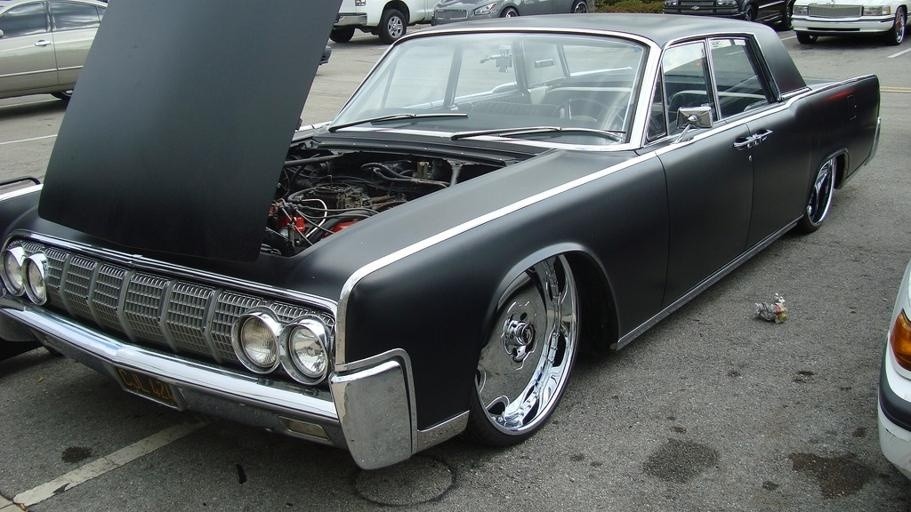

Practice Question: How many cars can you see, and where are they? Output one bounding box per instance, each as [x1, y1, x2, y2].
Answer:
[658, 0, 794, 29]
[428, 1, 597, 26]
[872, 252, 910, 487]
[329, 0, 436, 46]
[2, 1, 885, 476]
[0, 0, 109, 104]
[792, 1, 911, 48]
